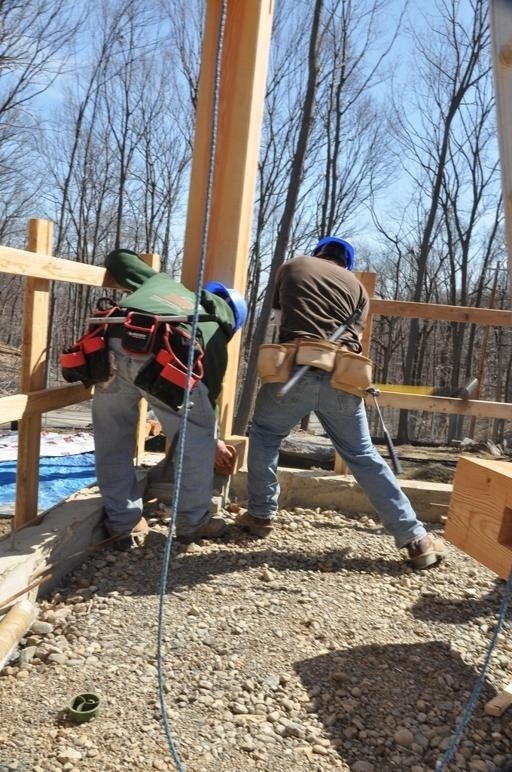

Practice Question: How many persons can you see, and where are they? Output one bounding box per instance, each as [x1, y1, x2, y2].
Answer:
[61, 249, 247, 549]
[232, 236, 441, 572]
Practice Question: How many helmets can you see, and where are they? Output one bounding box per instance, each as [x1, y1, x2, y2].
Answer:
[310, 235, 356, 270]
[206, 281, 249, 333]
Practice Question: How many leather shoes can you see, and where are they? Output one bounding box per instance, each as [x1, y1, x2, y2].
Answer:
[234, 512, 275, 537]
[114, 517, 149, 550]
[180, 518, 228, 544]
[408, 536, 446, 569]
